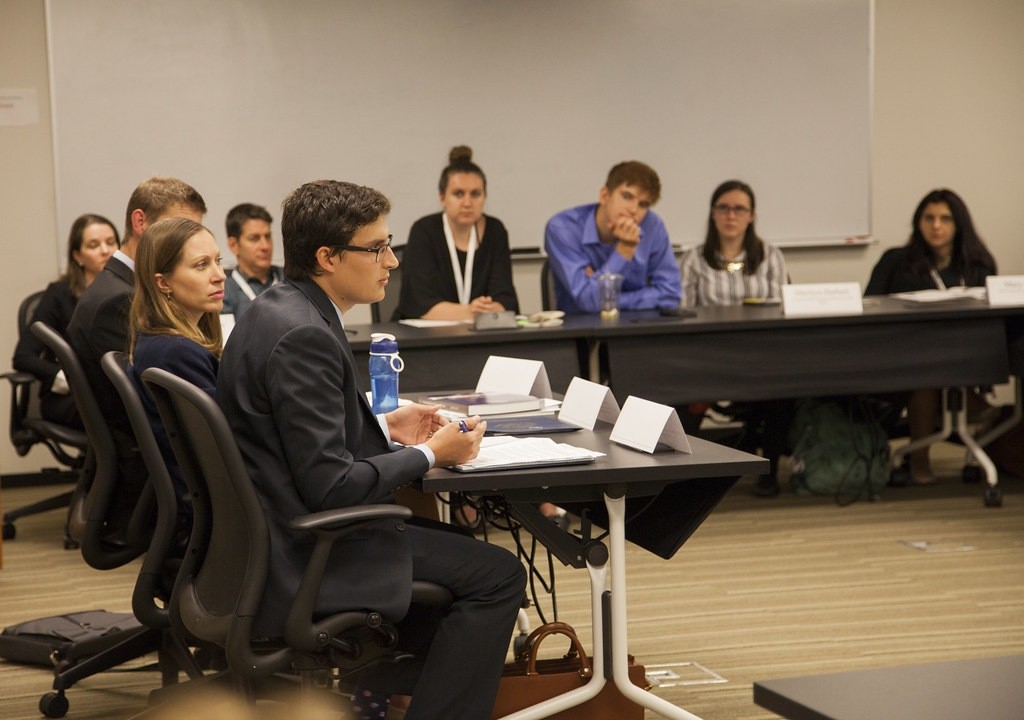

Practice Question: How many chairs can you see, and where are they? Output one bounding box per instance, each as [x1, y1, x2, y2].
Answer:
[1, 287, 452, 720]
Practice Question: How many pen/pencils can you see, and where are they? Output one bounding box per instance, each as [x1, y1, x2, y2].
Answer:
[459, 421, 468, 433]
[345, 329, 357, 335]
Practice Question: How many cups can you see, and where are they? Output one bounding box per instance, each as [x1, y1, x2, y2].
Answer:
[599, 274, 623, 321]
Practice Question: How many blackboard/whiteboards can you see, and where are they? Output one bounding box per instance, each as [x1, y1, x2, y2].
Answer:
[46, 0, 874, 277]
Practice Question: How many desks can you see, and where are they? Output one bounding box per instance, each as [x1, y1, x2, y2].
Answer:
[371, 387, 770, 720]
[339, 289, 1023, 506]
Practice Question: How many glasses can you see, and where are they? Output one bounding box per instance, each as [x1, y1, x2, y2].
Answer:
[326, 235, 393, 263]
[713, 203, 749, 217]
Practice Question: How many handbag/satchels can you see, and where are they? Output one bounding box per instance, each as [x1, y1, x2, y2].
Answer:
[1, 610, 145, 667]
[490, 622, 646, 720]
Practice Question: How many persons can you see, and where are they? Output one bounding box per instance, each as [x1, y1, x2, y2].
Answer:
[219, 205, 287, 326]
[861, 189, 1019, 486]
[217, 179, 527, 720]
[543, 161, 682, 313]
[679, 179, 792, 497]
[64, 177, 207, 519]
[12, 214, 121, 431]
[129, 216, 227, 403]
[393, 144, 519, 323]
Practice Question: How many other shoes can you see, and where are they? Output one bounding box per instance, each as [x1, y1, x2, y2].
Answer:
[755, 480, 779, 498]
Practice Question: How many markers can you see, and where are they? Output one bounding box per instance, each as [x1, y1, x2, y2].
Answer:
[846, 234, 870, 242]
[672, 244, 696, 249]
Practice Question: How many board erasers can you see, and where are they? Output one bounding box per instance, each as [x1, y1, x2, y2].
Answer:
[510, 248, 540, 255]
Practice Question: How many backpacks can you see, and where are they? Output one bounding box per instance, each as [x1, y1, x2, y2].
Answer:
[789, 400, 893, 504]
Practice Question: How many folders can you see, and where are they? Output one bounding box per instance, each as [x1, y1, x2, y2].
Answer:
[475, 415, 583, 437]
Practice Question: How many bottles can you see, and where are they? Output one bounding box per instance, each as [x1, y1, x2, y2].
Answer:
[368, 332, 405, 416]
